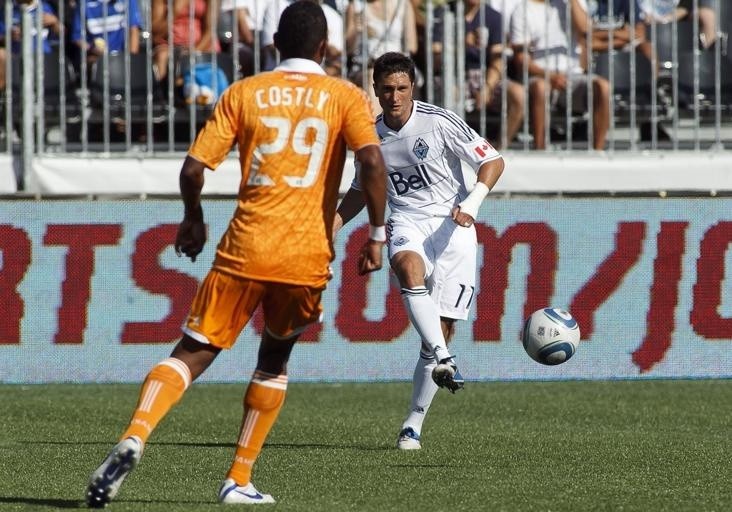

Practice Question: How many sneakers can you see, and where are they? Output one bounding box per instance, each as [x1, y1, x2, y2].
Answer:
[84, 435, 144, 508]
[219, 479, 275, 504]
[431, 356, 465, 393]
[396, 427, 421, 450]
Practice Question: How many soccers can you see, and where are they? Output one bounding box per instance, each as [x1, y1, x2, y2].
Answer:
[523, 308, 580, 366]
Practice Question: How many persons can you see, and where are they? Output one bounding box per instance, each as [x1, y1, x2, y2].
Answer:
[333, 50, 508, 450]
[572, 0, 732, 86]
[86, 2, 391, 509]
[1, 0, 611, 150]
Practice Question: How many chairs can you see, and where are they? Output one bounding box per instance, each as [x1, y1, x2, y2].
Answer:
[0, 22, 732, 147]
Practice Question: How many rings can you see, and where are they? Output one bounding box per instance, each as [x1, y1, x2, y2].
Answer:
[463, 220, 471, 228]
